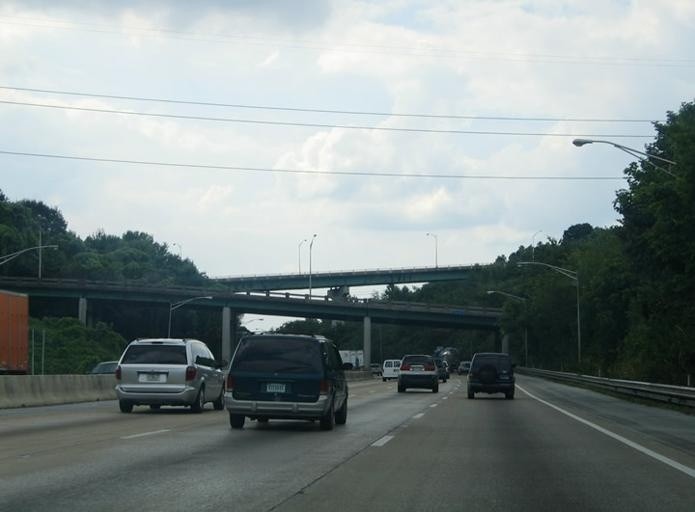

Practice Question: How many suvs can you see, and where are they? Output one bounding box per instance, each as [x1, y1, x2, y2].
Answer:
[371, 346, 519, 400]
[222, 328, 351, 429]
[112, 336, 226, 414]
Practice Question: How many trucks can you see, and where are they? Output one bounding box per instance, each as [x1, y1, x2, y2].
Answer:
[338, 347, 365, 371]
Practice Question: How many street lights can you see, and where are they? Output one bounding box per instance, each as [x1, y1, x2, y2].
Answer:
[1, 242, 62, 267]
[569, 137, 695, 206]
[167, 295, 213, 337]
[244, 318, 264, 325]
[517, 259, 586, 375]
[308, 232, 318, 300]
[173, 242, 183, 259]
[424, 231, 438, 268]
[297, 238, 307, 275]
[485, 289, 530, 368]
[532, 228, 543, 262]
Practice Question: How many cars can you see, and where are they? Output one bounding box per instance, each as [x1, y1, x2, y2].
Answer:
[88, 360, 121, 374]
